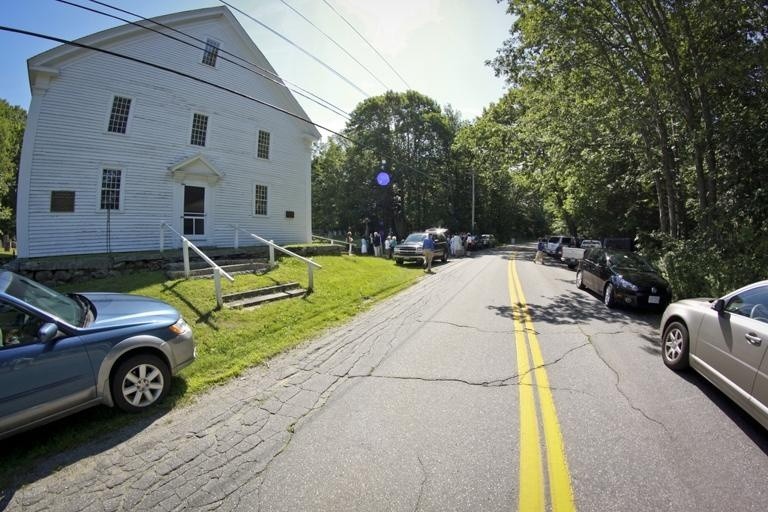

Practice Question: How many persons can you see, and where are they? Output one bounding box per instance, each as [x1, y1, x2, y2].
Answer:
[361, 232, 397, 258]
[447, 231, 475, 258]
[345, 231, 353, 254]
[533, 238, 543, 266]
[422, 233, 434, 271]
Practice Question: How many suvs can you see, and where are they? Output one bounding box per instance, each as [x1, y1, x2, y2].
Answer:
[393, 232, 450, 266]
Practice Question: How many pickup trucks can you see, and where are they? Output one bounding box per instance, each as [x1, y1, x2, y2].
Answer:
[561, 239, 603, 269]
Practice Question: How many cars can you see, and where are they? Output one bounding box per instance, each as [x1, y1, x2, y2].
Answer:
[603, 237, 634, 250]
[481, 234, 497, 248]
[659, 280, 768, 430]
[575, 247, 672, 310]
[538, 235, 574, 257]
[0, 268, 196, 440]
